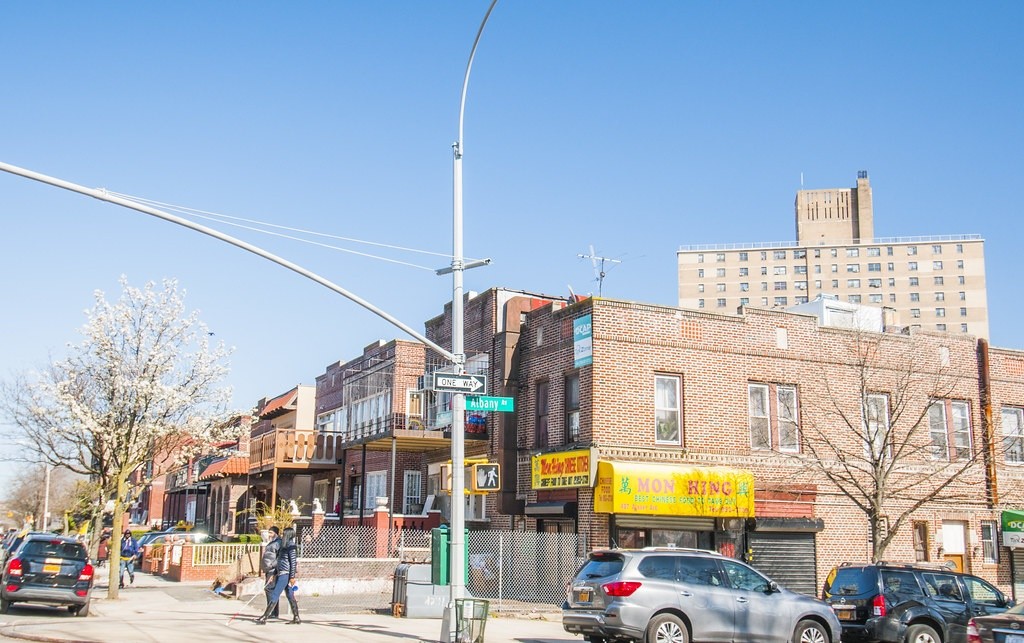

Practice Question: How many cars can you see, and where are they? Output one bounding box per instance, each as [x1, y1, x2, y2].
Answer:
[133, 520, 225, 568]
[967, 602, 1024, 643]
[0, 528, 54, 571]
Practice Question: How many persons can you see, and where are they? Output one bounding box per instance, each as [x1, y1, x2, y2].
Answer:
[259, 526, 282, 618]
[252, 528, 301, 625]
[940, 584, 961, 599]
[97, 536, 108, 566]
[118, 530, 139, 588]
[334, 496, 341, 516]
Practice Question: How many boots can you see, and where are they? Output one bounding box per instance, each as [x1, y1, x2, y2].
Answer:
[285, 599, 301, 624]
[252, 601, 277, 625]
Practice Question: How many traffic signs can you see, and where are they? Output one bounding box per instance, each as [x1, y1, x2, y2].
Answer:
[433, 371, 488, 395]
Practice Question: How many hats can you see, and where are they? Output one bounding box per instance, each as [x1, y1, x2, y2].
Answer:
[125, 530, 131, 534]
[269, 526, 279, 536]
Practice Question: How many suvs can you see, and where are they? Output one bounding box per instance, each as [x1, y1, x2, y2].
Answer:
[0, 534, 95, 618]
[821, 562, 1020, 643]
[561, 545, 845, 643]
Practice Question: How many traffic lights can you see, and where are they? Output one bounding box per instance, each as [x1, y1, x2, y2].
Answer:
[472, 464, 503, 494]
[441, 464, 452, 494]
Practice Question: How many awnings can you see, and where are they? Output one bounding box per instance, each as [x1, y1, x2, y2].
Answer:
[1001, 510, 1024, 547]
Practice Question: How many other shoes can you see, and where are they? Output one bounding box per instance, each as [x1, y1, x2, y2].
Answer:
[269, 613, 279, 619]
[130, 574, 134, 583]
[119, 583, 124, 589]
